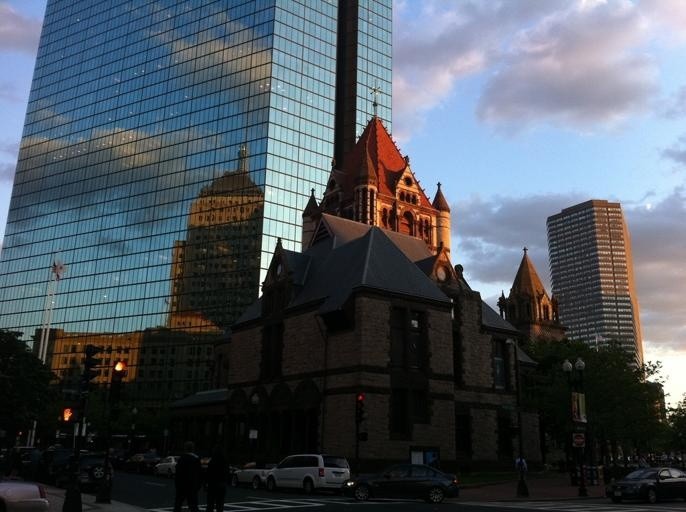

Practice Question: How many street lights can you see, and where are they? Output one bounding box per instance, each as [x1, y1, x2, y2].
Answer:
[561, 356, 588, 496]
[504, 336, 530, 498]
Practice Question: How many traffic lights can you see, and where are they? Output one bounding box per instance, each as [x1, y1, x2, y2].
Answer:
[62, 407, 80, 424]
[355, 393, 365, 424]
[85, 343, 101, 380]
[109, 359, 128, 404]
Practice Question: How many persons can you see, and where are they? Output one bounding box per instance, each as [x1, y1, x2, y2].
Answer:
[169, 438, 204, 510]
[199, 439, 228, 511]
[514, 456, 527, 480]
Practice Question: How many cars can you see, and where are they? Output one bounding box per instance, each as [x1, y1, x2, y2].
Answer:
[0, 480, 51, 512]
[231, 454, 460, 504]
[13, 446, 106, 487]
[607, 467, 686, 504]
[125, 452, 184, 479]
